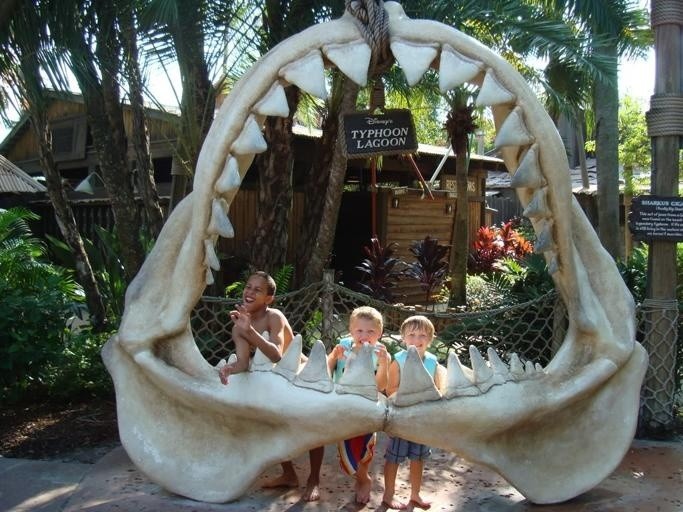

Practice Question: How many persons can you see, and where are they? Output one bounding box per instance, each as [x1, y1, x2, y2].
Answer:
[386, 316, 447, 509]
[219, 269, 324, 501]
[328, 306, 391, 504]
